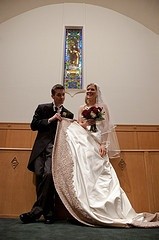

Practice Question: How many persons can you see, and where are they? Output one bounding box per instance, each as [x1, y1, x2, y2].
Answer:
[78, 82, 109, 226]
[20, 84, 74, 224]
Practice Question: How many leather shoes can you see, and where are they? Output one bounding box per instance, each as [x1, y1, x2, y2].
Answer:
[44, 217, 56, 224]
[19, 212, 39, 223]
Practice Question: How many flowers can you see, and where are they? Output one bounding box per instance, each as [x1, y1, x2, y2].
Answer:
[81, 106, 105, 132]
